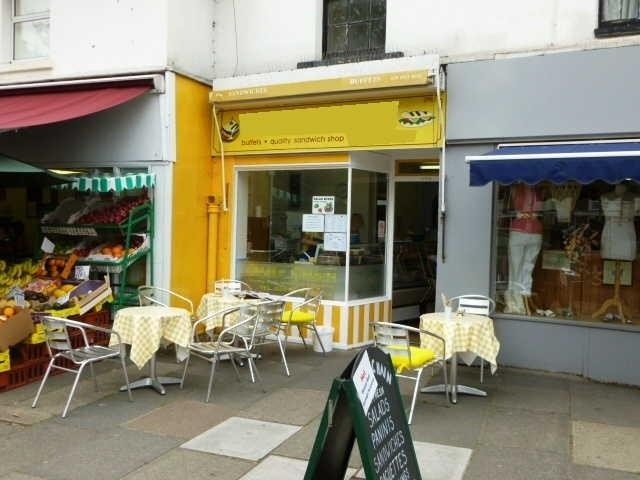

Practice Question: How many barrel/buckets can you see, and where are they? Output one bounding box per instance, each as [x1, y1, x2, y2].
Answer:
[313, 327, 333, 353]
[350, 248, 369, 265]
[316, 255, 340, 265]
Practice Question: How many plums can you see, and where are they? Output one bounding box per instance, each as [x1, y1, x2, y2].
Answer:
[114, 235, 144, 249]
[74, 240, 104, 257]
[8, 290, 47, 302]
[75, 195, 143, 224]
[30, 312, 53, 325]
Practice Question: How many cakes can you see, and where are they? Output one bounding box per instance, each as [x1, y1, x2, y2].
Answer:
[221, 115, 240, 142]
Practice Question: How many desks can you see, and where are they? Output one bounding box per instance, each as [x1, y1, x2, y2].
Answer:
[413, 312, 503, 405]
[193, 293, 270, 368]
[105, 306, 195, 398]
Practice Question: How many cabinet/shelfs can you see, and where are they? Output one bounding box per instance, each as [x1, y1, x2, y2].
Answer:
[348, 240, 432, 324]
[38, 191, 157, 324]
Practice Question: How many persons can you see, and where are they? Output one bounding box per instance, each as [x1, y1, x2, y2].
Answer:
[505, 184, 546, 295]
[427, 255, 437, 263]
[601, 183, 640, 261]
[302, 233, 323, 261]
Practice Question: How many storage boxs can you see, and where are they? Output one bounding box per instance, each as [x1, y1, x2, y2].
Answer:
[0, 277, 112, 354]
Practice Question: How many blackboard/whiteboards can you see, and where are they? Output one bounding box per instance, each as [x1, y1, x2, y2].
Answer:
[342, 348, 423, 480]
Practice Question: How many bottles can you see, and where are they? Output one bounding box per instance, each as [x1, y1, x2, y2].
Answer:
[255, 204, 263, 217]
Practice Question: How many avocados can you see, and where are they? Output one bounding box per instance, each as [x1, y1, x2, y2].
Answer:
[34, 303, 62, 311]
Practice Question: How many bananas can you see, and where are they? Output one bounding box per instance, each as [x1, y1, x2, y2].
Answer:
[0, 257, 40, 298]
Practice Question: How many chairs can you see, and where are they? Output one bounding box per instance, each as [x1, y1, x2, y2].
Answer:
[178, 303, 264, 404]
[137, 284, 198, 318]
[223, 299, 291, 381]
[272, 285, 327, 377]
[212, 278, 266, 355]
[368, 318, 452, 428]
[440, 293, 497, 385]
[30, 316, 135, 420]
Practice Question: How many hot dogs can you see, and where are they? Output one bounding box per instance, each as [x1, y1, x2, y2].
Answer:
[399, 111, 434, 127]
